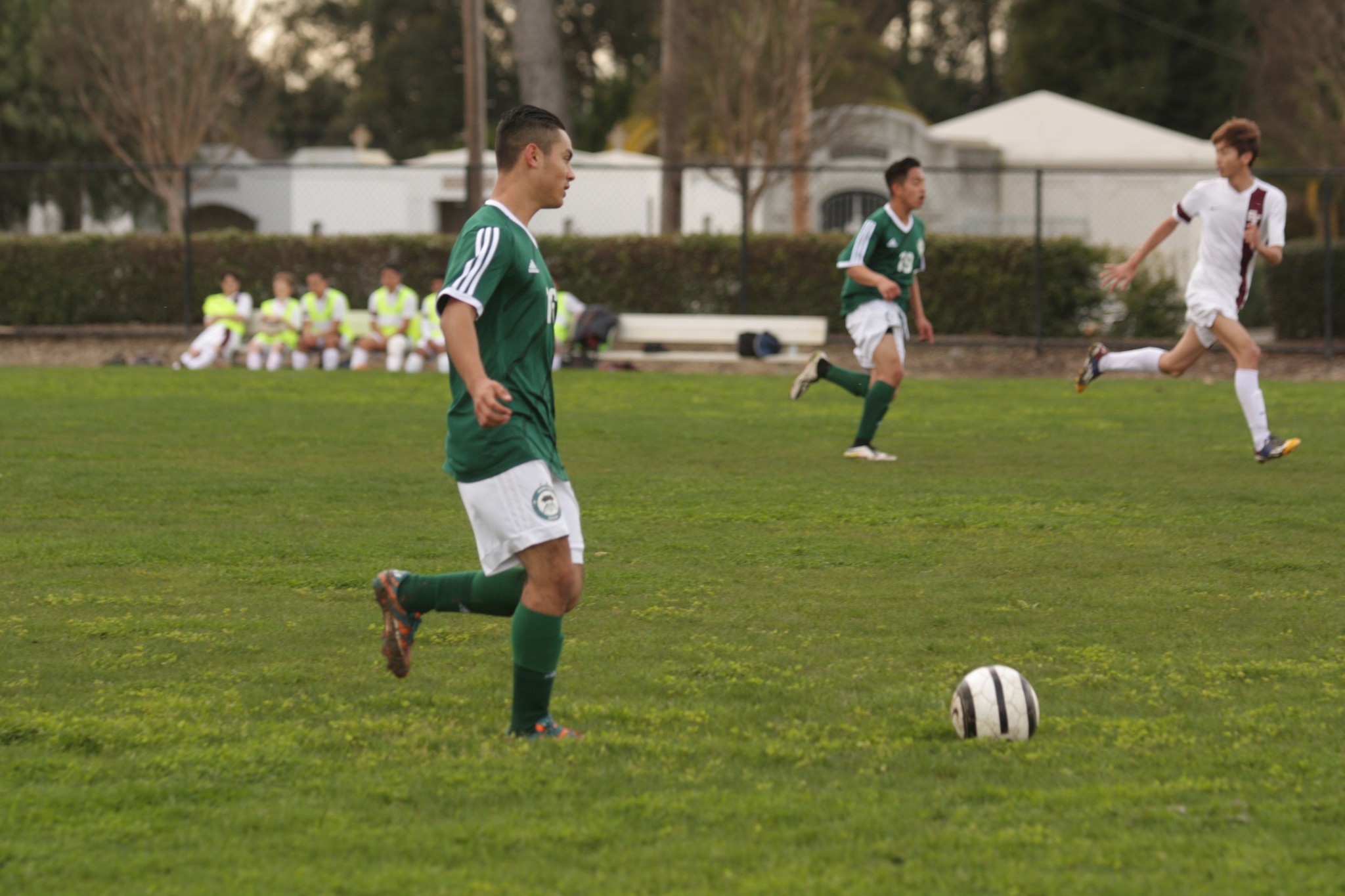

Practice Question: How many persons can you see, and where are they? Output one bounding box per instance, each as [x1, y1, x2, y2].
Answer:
[788, 156, 934, 456]
[372, 103, 586, 740]
[171, 263, 450, 373]
[551, 275, 585, 371]
[1075, 118, 1302, 464]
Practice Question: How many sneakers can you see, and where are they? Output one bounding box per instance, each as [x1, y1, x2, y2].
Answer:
[1076, 342, 1110, 391]
[1255, 435, 1302, 463]
[373, 568, 421, 677]
[791, 351, 830, 400]
[534, 715, 583, 739]
[843, 445, 898, 462]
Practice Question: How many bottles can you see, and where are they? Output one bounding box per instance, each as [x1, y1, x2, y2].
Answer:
[171, 361, 182, 370]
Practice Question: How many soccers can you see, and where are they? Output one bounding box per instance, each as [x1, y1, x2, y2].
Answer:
[950, 664, 1040, 743]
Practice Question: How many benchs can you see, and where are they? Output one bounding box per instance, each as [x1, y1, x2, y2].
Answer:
[220, 306, 829, 377]
